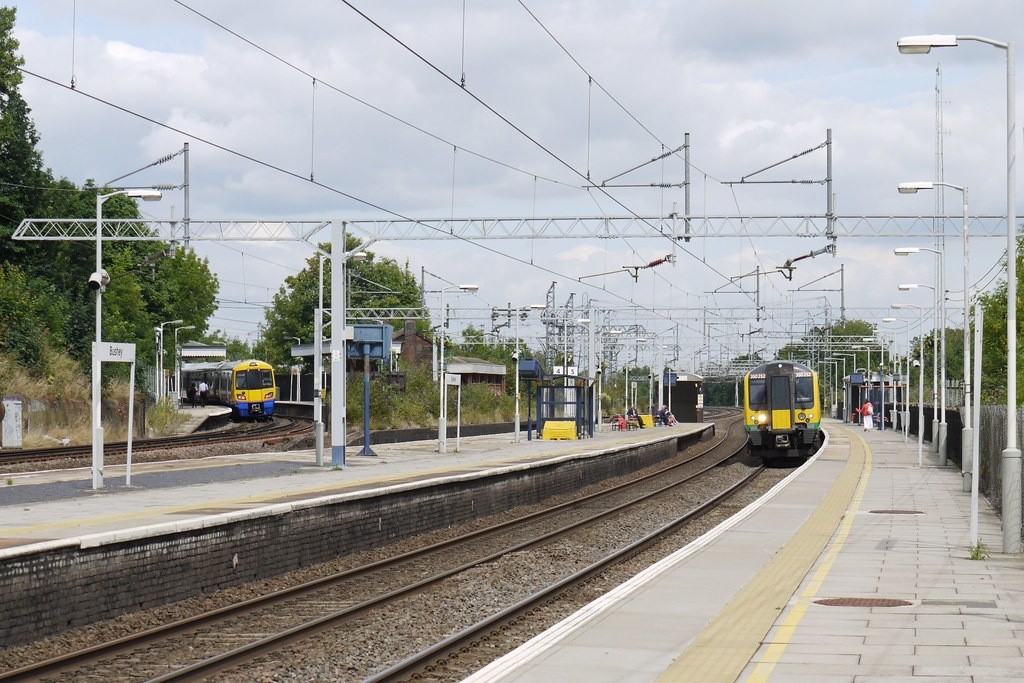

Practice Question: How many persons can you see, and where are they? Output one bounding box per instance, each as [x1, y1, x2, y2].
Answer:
[857, 399, 874, 432]
[188, 383, 198, 408]
[664, 407, 679, 425]
[627, 404, 646, 429]
[198, 380, 208, 407]
[658, 405, 673, 426]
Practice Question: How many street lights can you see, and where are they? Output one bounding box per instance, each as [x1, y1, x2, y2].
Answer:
[896, 283, 939, 455]
[564, 318, 590, 416]
[899, 178, 973, 496]
[818, 335, 897, 432]
[599, 330, 621, 431]
[92, 183, 164, 489]
[513, 303, 546, 442]
[624, 338, 646, 421]
[315, 251, 368, 465]
[437, 282, 479, 451]
[891, 302, 926, 447]
[873, 328, 906, 436]
[895, 244, 949, 468]
[898, 29, 1023, 552]
[883, 317, 911, 439]
[648, 344, 669, 415]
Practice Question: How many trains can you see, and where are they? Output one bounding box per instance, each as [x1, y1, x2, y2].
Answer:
[181, 359, 277, 420]
[743, 359, 822, 468]
